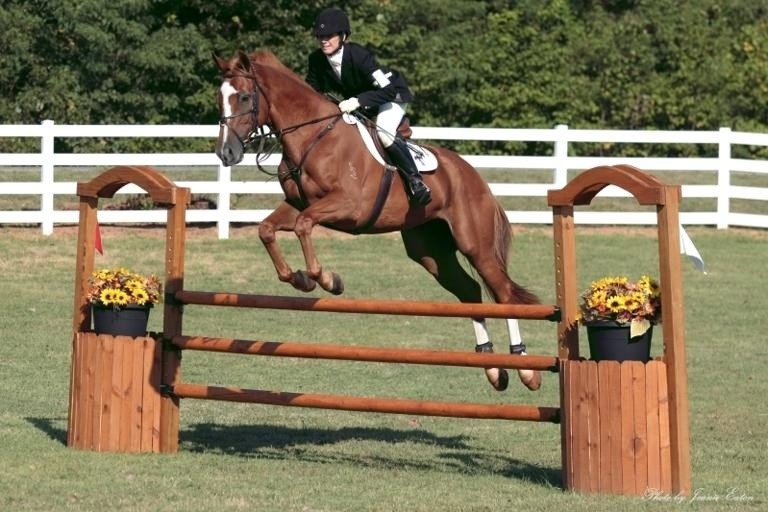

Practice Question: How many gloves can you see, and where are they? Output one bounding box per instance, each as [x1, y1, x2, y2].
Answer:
[339, 97, 360, 114]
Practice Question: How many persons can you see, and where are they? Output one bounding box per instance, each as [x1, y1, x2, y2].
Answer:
[303, 8, 433, 211]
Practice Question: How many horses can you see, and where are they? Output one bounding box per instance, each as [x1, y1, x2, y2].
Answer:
[211, 47, 543, 391]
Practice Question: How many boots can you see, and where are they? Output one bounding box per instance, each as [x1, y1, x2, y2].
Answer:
[386, 135, 432, 208]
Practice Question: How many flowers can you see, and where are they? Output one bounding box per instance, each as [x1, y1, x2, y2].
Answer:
[575, 274, 663, 329]
[84, 264, 162, 310]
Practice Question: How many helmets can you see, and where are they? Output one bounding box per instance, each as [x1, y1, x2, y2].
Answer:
[311, 8, 350, 36]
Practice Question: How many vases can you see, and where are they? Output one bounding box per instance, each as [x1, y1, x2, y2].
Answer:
[584, 319, 654, 366]
[91, 301, 151, 341]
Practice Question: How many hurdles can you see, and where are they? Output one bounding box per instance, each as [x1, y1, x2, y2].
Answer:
[66, 165, 692, 498]
